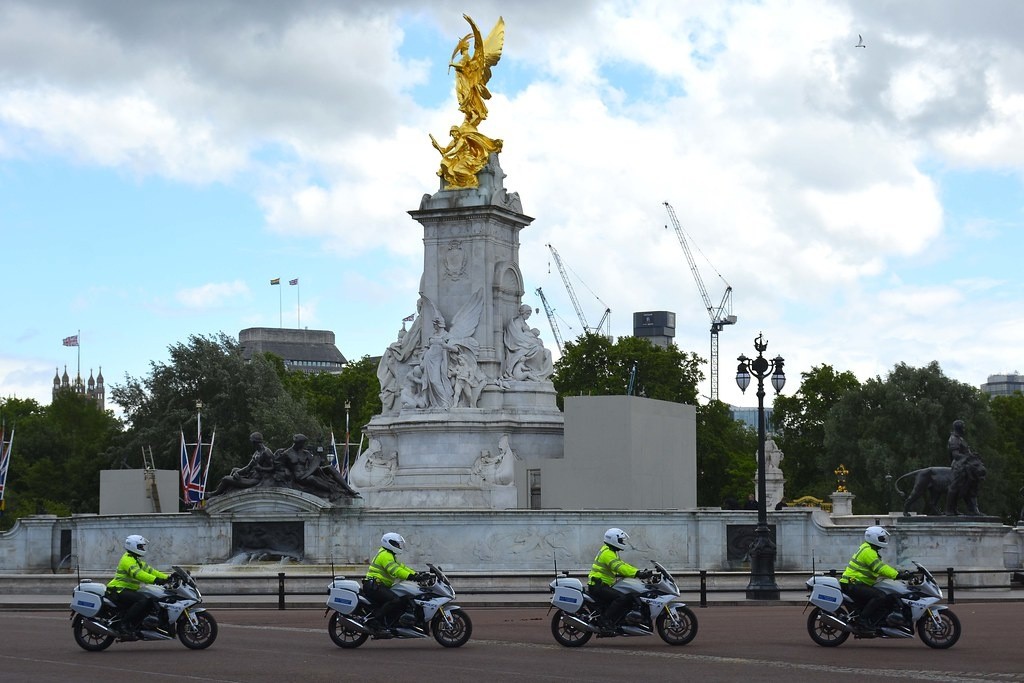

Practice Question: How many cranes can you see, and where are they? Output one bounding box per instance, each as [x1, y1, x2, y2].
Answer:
[545, 242, 610, 342]
[535, 287, 579, 358]
[664, 201, 737, 401]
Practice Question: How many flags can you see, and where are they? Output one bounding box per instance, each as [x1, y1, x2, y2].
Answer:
[63, 335, 79, 346]
[270, 278, 280, 285]
[0, 430, 12, 500]
[329, 415, 364, 486]
[289, 279, 299, 285]
[181, 430, 215, 506]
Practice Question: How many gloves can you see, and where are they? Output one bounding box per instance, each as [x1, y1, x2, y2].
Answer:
[408, 573, 423, 581]
[154, 577, 170, 585]
[896, 572, 914, 580]
[638, 568, 653, 578]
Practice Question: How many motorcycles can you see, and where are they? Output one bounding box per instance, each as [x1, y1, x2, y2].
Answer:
[803, 549, 961, 649]
[69, 555, 218, 653]
[546, 551, 699, 648]
[324, 554, 473, 649]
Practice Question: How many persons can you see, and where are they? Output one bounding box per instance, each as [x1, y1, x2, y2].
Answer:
[432, 126, 502, 190]
[449, 14, 505, 129]
[765, 432, 778, 468]
[378, 299, 474, 408]
[588, 527, 646, 630]
[949, 419, 979, 491]
[106, 535, 171, 635]
[272, 434, 362, 498]
[363, 533, 424, 629]
[349, 440, 398, 488]
[208, 432, 274, 498]
[471, 435, 514, 486]
[504, 305, 553, 381]
[839, 527, 909, 632]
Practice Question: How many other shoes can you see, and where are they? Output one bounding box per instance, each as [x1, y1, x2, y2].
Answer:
[615, 625, 630, 634]
[116, 623, 133, 635]
[370, 622, 387, 630]
[854, 623, 875, 631]
[599, 621, 618, 630]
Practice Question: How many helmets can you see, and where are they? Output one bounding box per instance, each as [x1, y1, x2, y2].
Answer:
[604, 527, 630, 551]
[865, 526, 891, 548]
[123, 534, 149, 557]
[380, 532, 404, 554]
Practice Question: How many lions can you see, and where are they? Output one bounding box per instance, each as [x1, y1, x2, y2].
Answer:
[893, 452, 989, 517]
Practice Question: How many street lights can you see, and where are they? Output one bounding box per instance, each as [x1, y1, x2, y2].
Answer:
[735, 330, 787, 600]
[886, 472, 893, 512]
